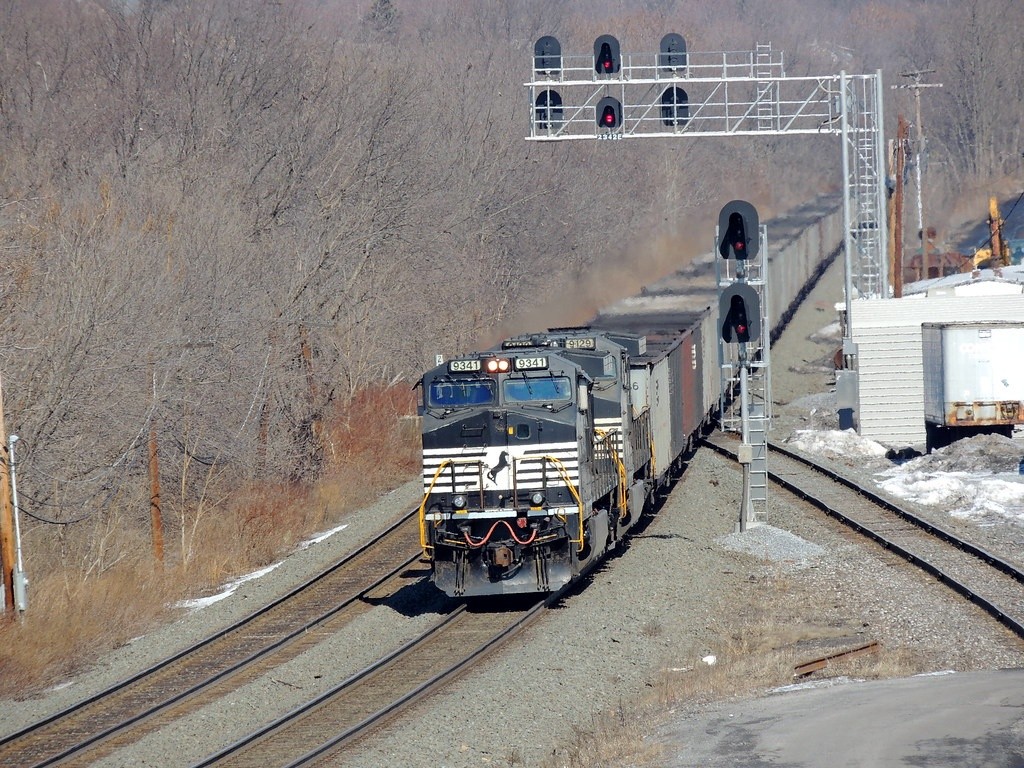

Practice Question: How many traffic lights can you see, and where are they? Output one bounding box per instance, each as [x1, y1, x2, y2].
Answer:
[716, 199, 759, 260]
[718, 283, 761, 343]
[597, 97, 622, 128]
[593, 34, 620, 74]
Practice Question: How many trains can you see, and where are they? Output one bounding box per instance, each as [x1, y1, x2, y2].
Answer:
[412, 171, 888, 602]
